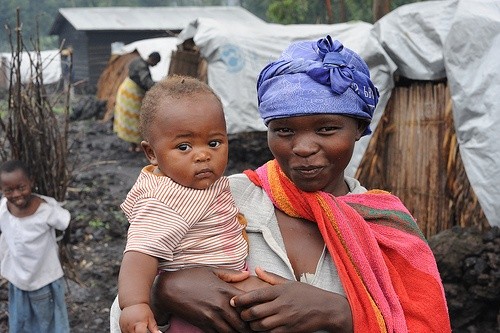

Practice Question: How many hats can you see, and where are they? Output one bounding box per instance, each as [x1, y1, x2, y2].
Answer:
[256, 35, 379, 136]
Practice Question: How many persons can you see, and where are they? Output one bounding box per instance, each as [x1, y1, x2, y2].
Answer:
[115, 74, 287, 333]
[113, 52, 162, 152]
[0, 159, 72, 333]
[116, 37, 452, 333]
